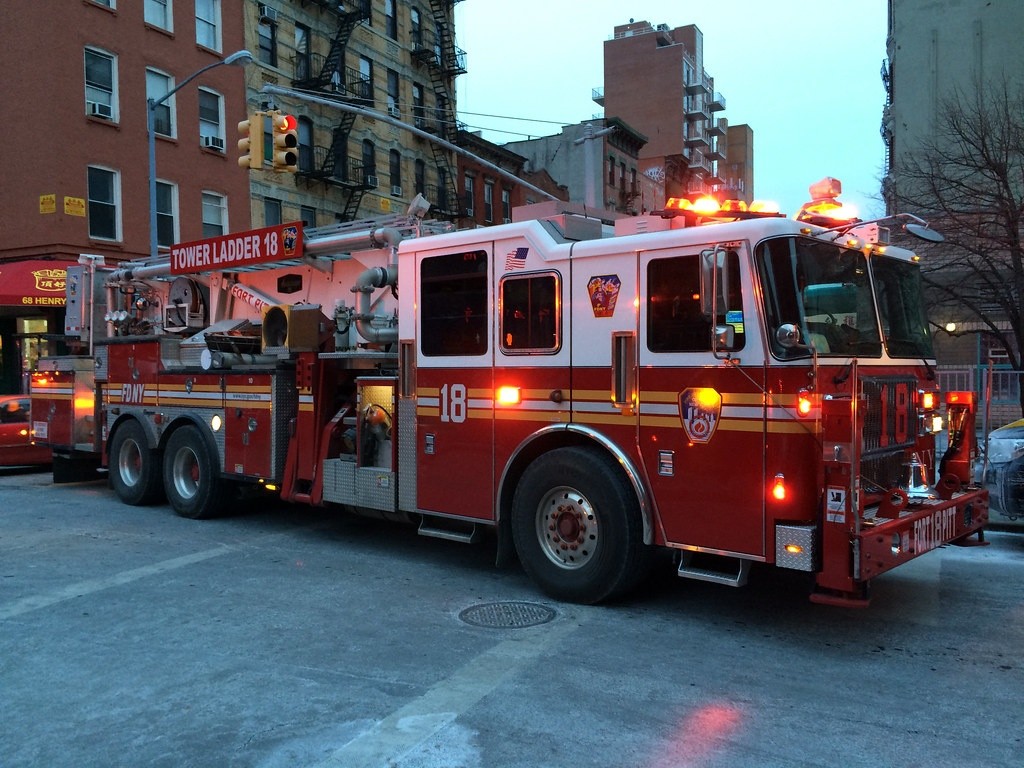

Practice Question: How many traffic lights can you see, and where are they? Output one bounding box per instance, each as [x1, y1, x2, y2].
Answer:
[237, 113, 262, 171]
[272, 112, 300, 173]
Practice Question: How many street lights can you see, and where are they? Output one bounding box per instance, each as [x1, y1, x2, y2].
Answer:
[146, 49, 254, 256]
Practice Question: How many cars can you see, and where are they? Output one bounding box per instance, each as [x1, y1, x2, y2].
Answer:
[978, 426, 1024, 520]
[0, 396, 58, 465]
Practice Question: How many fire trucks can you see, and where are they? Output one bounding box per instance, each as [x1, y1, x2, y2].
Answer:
[30, 184, 991, 617]
